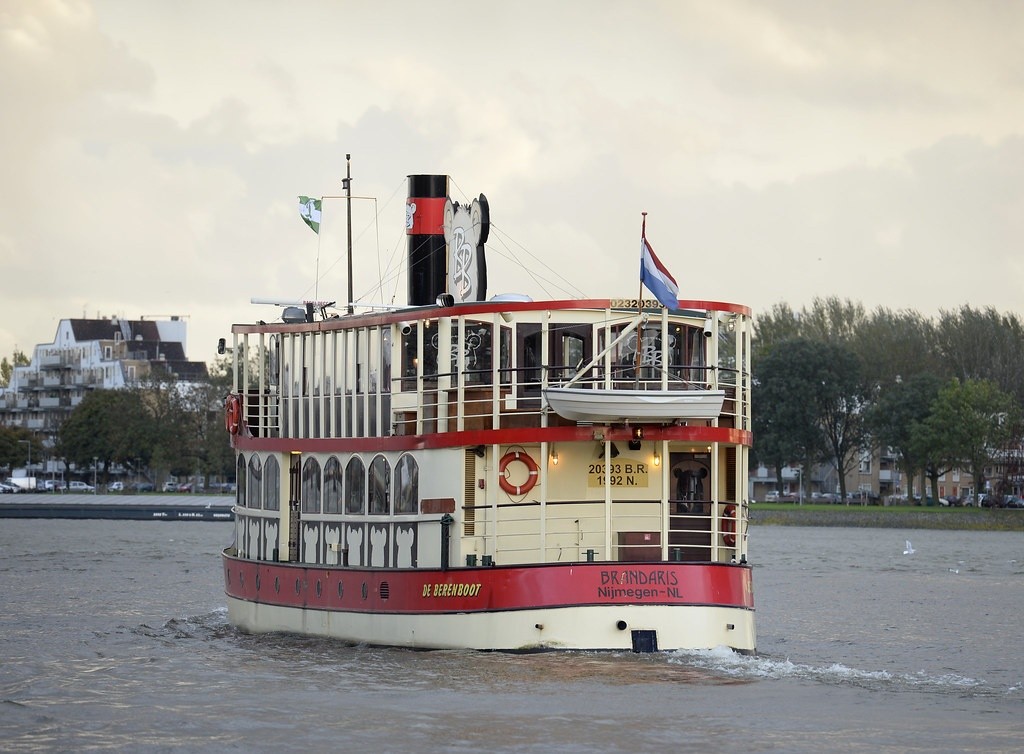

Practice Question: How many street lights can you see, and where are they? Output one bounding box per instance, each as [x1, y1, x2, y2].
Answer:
[93, 457, 99, 495]
[134, 458, 142, 494]
[18, 439, 31, 492]
[61, 456, 65, 494]
[52, 456, 57, 493]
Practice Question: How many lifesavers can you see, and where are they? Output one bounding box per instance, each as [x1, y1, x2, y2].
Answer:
[223, 394, 240, 436]
[498, 452, 539, 496]
[721, 504, 736, 547]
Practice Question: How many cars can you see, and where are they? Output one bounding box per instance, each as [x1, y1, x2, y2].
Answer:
[0, 476, 96, 495]
[137, 482, 156, 492]
[107, 481, 124, 492]
[162, 476, 235, 493]
[765, 487, 1024, 508]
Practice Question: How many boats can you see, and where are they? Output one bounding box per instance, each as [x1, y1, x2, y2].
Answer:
[541, 383, 725, 425]
[221, 154, 760, 659]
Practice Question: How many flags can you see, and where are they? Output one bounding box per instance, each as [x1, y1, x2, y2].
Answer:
[640, 220, 680, 311]
[298, 195, 322, 234]
